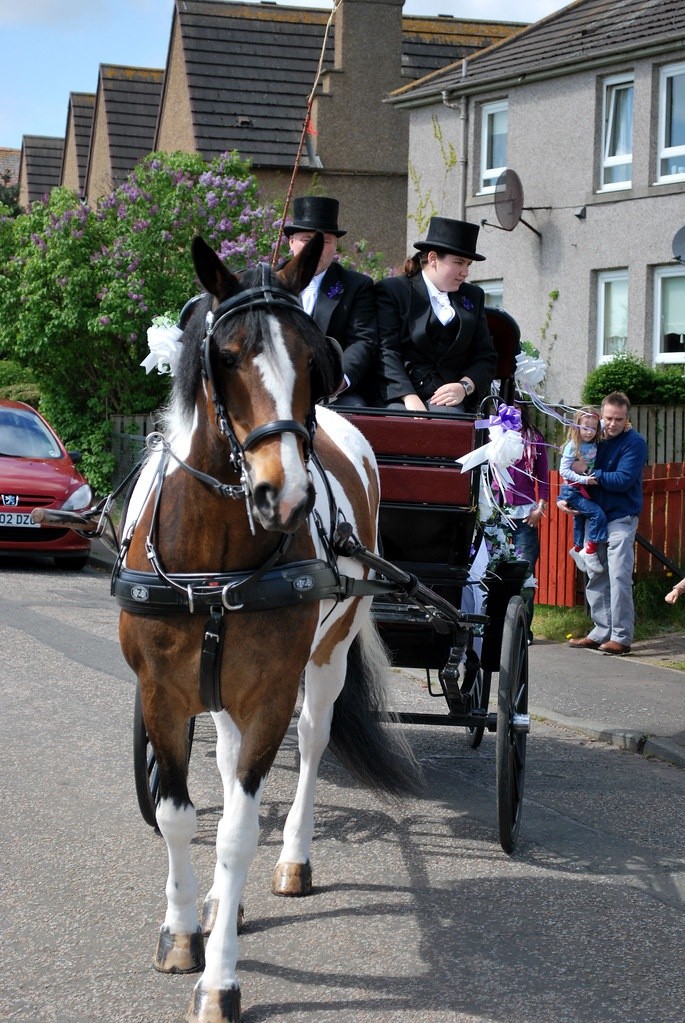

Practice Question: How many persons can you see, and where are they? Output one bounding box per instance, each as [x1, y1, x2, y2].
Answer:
[557, 391, 646, 654]
[255, 194, 378, 411]
[664, 578, 684, 605]
[371, 216, 494, 421]
[560, 407, 632, 571]
[484, 393, 548, 645]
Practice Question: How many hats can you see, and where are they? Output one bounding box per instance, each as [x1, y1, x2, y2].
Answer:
[413, 217, 487, 261]
[283, 196, 347, 239]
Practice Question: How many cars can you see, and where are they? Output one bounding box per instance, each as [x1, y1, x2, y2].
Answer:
[0, 397, 98, 572]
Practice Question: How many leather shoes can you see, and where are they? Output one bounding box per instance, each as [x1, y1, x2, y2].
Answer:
[569, 636, 601, 648]
[598, 641, 630, 654]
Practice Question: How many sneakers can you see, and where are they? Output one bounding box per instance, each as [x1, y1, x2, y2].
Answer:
[569, 546, 587, 572]
[579, 547, 603, 573]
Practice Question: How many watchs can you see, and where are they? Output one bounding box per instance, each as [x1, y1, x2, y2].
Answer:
[458, 380, 472, 395]
[538, 502, 547, 510]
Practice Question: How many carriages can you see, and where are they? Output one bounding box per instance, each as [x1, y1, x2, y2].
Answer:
[28, 229, 542, 1023]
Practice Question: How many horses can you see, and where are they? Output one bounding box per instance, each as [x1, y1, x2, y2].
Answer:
[118, 234, 380, 1023]
